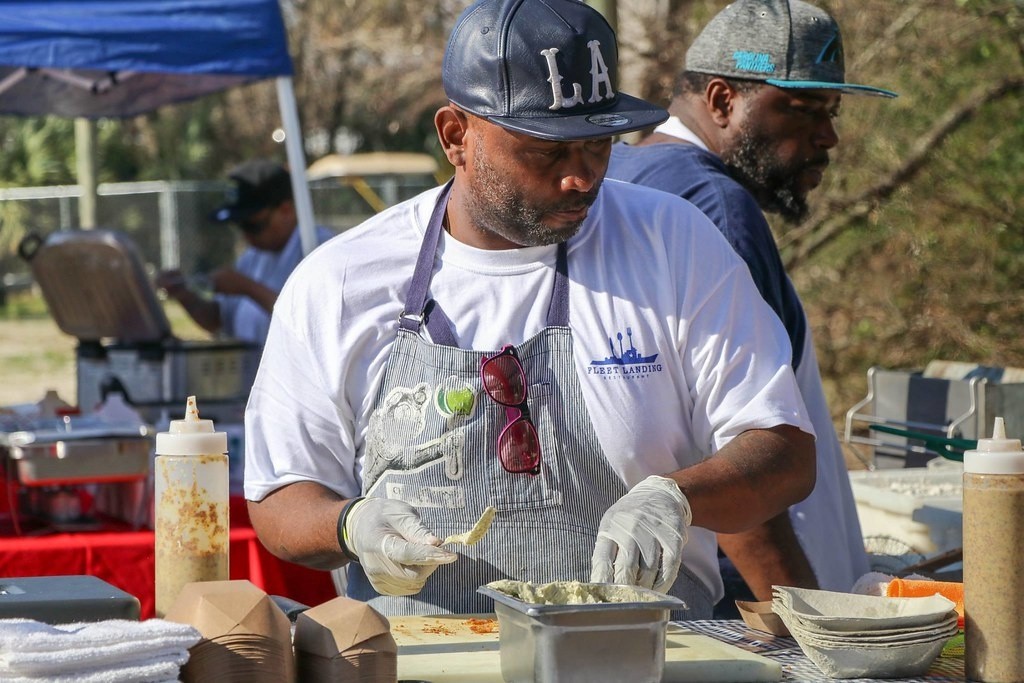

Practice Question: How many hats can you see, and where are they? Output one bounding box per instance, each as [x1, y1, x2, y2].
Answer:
[440, 0, 672, 142]
[683, 1, 898, 99]
[230, 161, 293, 213]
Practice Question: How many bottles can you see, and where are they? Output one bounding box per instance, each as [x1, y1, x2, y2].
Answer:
[962, 414, 1024, 683]
[154, 396, 228, 619]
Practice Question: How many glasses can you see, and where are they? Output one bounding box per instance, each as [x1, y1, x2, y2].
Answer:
[229, 210, 274, 233]
[481, 344, 542, 478]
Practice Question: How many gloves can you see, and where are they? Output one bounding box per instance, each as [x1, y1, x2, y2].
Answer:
[590, 474, 694, 595]
[347, 496, 459, 598]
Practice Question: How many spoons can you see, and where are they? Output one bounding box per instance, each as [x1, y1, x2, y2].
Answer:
[437, 507, 495, 547]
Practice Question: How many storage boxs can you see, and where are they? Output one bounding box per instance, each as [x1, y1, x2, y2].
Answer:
[18, 228, 261, 429]
[847, 466, 963, 554]
[0, 430, 152, 538]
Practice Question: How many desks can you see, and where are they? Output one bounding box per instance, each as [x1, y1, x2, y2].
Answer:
[0, 492, 337, 608]
[150, 614, 965, 683]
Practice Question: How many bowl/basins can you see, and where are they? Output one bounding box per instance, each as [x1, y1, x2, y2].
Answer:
[770, 584, 960, 680]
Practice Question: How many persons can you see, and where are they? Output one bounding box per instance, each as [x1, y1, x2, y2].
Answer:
[240, 0, 821, 624]
[601, 2, 877, 625]
[153, 160, 336, 347]
